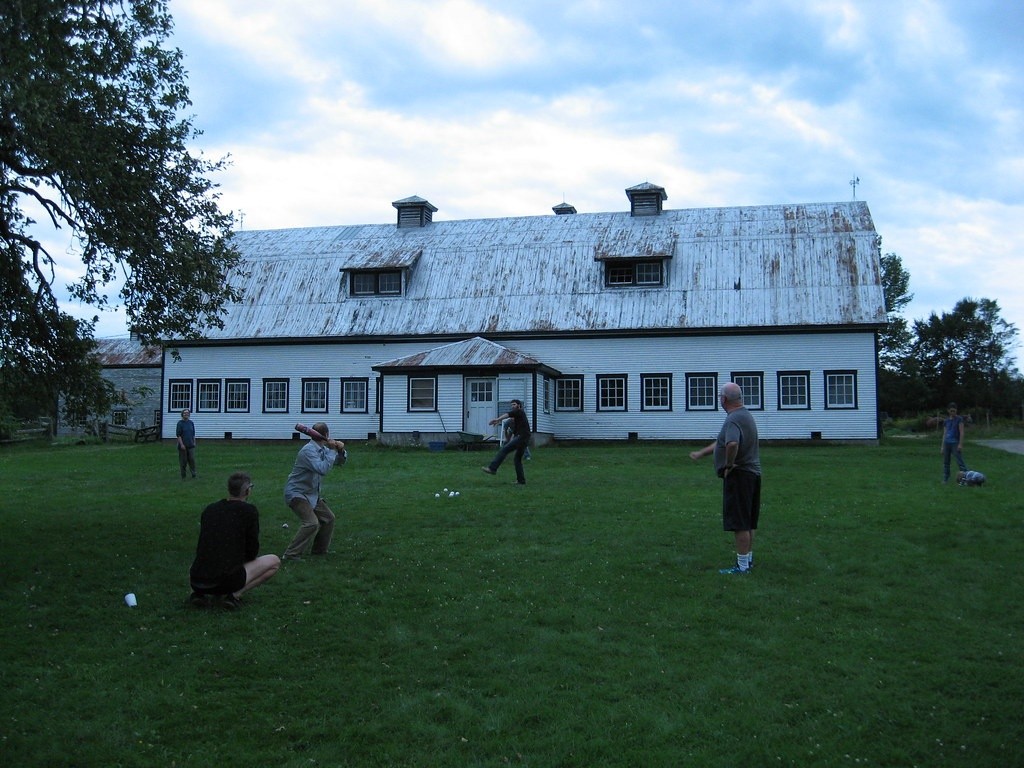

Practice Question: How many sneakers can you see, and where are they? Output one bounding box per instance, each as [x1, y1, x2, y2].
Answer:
[190, 591, 208, 605]
[219, 593, 238, 606]
[720, 565, 751, 575]
[481, 467, 496, 475]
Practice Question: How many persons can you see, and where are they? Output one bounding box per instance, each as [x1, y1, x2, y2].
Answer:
[481, 399, 532, 485]
[503, 412, 532, 461]
[689, 381, 763, 576]
[955, 471, 986, 488]
[189, 473, 283, 612]
[175, 408, 197, 483]
[281, 421, 348, 564]
[938, 402, 968, 487]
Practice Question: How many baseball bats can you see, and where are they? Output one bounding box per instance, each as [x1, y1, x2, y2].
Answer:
[294, 423, 341, 447]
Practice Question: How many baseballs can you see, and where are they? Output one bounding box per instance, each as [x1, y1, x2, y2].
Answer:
[282, 523, 288, 529]
[450, 491, 454, 496]
[448, 494, 453, 499]
[443, 488, 448, 492]
[455, 491, 461, 496]
[434, 493, 440, 498]
